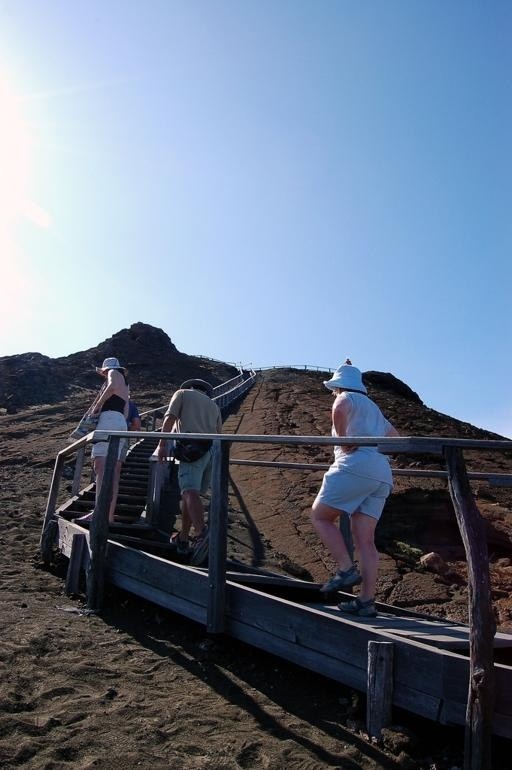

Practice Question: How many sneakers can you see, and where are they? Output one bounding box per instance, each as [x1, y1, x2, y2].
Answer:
[338, 596, 376, 616]
[319, 564, 363, 593]
[76, 510, 94, 521]
[170, 532, 189, 554]
[190, 527, 209, 566]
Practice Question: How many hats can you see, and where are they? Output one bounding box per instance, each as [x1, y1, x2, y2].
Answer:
[96, 357, 129, 376]
[323, 364, 368, 394]
[179, 378, 213, 395]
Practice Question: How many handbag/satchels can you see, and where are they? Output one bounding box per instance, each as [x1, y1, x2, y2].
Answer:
[174, 439, 213, 463]
[69, 410, 99, 439]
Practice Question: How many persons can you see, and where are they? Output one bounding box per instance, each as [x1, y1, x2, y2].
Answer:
[70, 357, 130, 524]
[156, 377, 223, 566]
[113, 398, 141, 514]
[309, 363, 403, 618]
